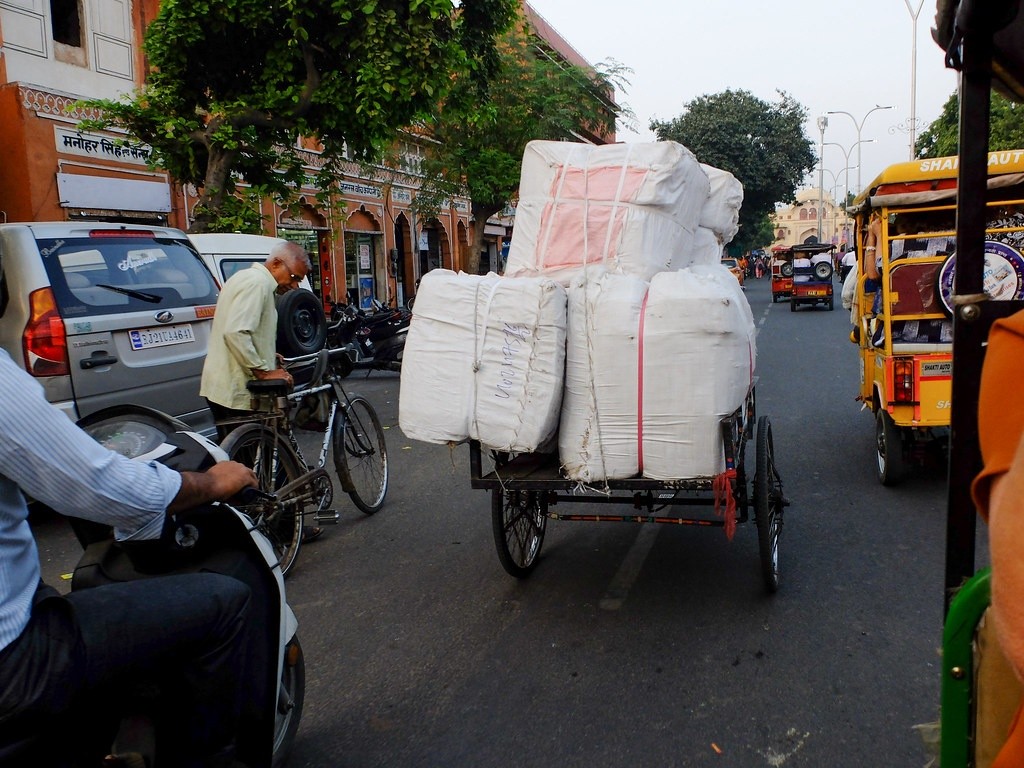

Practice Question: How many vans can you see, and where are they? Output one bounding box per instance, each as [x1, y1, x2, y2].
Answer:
[59, 231, 327, 385]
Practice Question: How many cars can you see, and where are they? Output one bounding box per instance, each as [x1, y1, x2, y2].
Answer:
[1, 220, 226, 451]
[721, 257, 744, 286]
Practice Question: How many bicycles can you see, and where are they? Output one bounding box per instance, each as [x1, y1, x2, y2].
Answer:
[209, 344, 388, 585]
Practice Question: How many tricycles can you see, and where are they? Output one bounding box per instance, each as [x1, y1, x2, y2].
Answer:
[469, 383, 785, 595]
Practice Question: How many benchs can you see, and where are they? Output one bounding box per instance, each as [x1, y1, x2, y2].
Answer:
[63, 268, 197, 306]
[791, 267, 832, 285]
[858, 272, 877, 351]
[772, 265, 792, 279]
[939, 566, 1024, 768]
[873, 253, 954, 356]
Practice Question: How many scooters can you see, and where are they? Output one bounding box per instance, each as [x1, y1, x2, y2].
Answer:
[325, 280, 423, 380]
[790, 242, 837, 311]
[16, 404, 306, 768]
[847, 149, 1024, 487]
[770, 245, 794, 303]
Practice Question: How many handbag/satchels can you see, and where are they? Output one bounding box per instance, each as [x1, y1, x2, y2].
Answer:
[758, 264, 763, 270]
[296, 349, 332, 431]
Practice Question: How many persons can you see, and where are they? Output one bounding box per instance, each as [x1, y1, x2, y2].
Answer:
[738, 251, 835, 283]
[835, 206, 1024, 349]
[0, 347, 276, 768]
[199, 240, 324, 549]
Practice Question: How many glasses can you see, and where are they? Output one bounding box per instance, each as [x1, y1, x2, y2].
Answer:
[278, 258, 302, 283]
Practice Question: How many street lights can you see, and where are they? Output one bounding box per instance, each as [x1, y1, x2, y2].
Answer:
[814, 106, 895, 242]
[817, 116, 828, 241]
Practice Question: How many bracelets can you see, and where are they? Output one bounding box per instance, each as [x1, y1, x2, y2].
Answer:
[866, 246, 876, 251]
